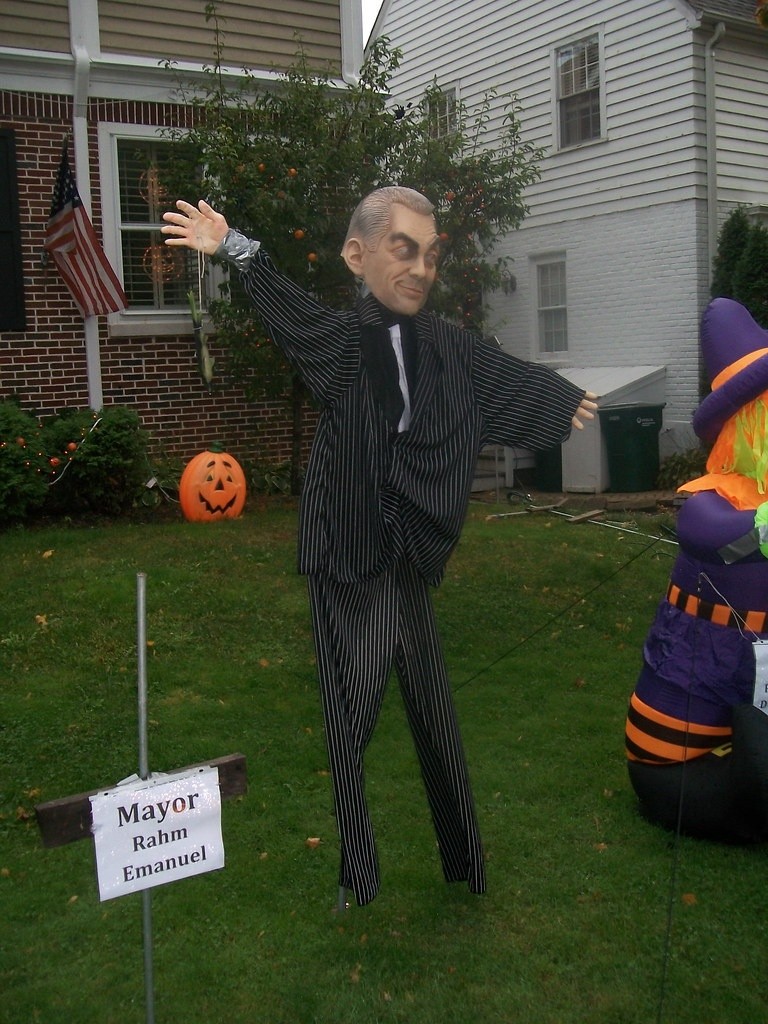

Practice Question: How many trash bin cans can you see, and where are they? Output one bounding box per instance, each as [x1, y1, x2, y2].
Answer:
[596, 401, 666, 494]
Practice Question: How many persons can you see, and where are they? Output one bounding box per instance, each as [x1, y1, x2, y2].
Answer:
[156, 185, 597, 915]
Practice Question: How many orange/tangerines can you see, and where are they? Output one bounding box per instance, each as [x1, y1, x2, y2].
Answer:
[236, 157, 317, 262]
[419, 172, 485, 242]
[16, 436, 79, 466]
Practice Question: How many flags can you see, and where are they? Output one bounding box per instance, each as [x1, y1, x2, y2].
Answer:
[45, 143, 128, 323]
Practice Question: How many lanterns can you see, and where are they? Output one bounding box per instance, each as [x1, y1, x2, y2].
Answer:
[177, 435, 249, 523]
